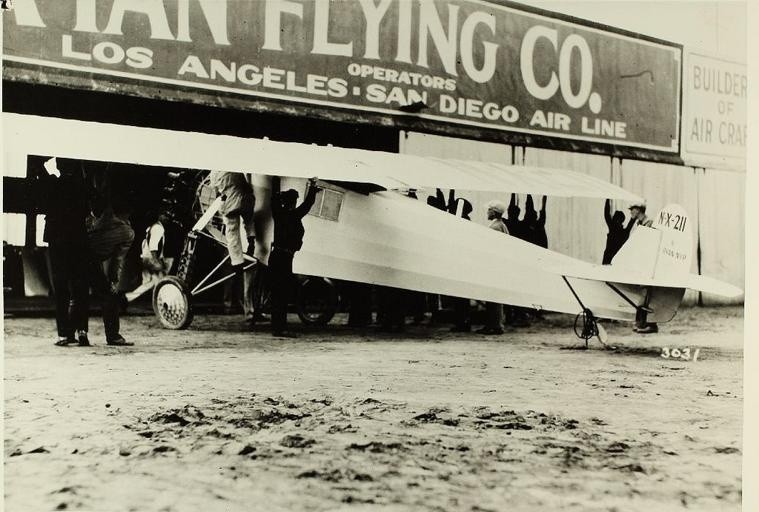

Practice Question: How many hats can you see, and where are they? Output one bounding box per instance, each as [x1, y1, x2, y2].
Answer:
[628, 201, 647, 209]
[486, 200, 505, 215]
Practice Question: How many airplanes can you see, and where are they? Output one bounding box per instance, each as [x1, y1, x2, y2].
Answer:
[2, 112, 743, 334]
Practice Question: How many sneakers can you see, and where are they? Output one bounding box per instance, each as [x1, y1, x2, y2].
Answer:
[632, 322, 659, 334]
[245, 245, 255, 256]
[234, 267, 244, 278]
[107, 336, 135, 346]
[350, 314, 547, 335]
[53, 330, 90, 346]
[272, 328, 297, 338]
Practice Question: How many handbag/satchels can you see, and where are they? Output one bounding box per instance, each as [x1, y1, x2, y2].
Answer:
[142, 255, 167, 273]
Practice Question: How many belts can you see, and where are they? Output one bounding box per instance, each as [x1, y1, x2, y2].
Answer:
[273, 246, 295, 256]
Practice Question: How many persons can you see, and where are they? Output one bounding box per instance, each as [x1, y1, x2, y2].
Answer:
[32, 155, 92, 346]
[267, 174, 323, 334]
[210, 170, 256, 281]
[68, 163, 141, 346]
[347, 185, 659, 333]
